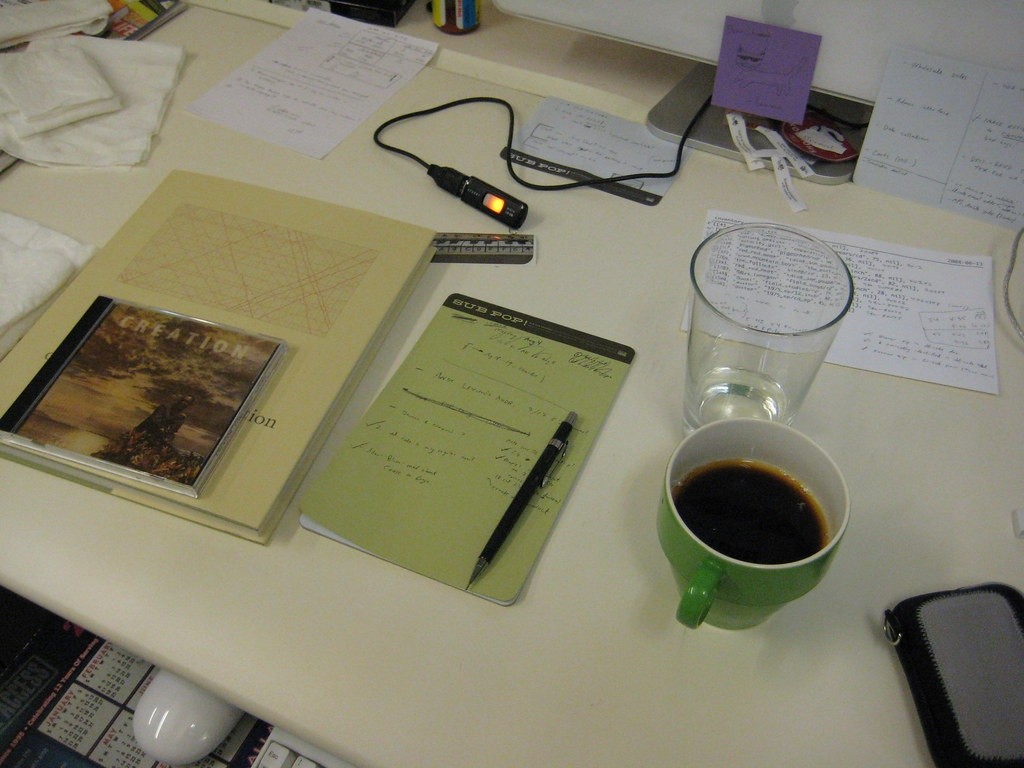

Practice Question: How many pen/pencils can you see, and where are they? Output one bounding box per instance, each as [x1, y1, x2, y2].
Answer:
[465, 410, 578, 591]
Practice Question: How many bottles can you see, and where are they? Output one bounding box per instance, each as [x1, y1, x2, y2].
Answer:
[431, 0, 481, 35]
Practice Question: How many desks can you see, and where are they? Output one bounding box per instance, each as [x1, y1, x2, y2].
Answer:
[0, 4, 1024, 768]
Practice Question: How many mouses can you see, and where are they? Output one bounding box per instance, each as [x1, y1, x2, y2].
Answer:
[133, 668, 246, 766]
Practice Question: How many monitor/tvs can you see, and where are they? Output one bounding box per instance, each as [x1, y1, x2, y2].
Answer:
[491, 1, 1024, 190]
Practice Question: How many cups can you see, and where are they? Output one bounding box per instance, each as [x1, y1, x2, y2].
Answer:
[656, 416, 850, 629]
[672, 223, 855, 451]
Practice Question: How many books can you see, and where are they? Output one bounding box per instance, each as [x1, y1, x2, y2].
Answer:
[71, 0, 187, 41]
[0, 167, 440, 545]
[299, 293, 635, 606]
